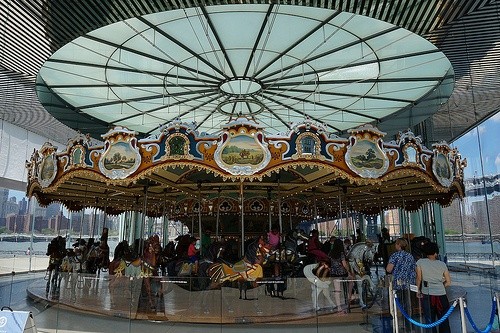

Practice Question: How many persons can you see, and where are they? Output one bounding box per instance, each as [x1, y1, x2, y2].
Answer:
[201, 227, 212, 257]
[307, 229, 355, 312]
[257, 225, 283, 281]
[26, 248, 34, 255]
[187, 237, 201, 276]
[386, 238, 417, 329]
[416, 241, 451, 333]
[352, 228, 390, 258]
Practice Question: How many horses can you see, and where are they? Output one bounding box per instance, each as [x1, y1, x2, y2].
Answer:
[199, 238, 264, 301]
[45, 238, 67, 294]
[301, 241, 380, 310]
[168, 239, 233, 284]
[264, 228, 309, 278]
[109, 235, 166, 310]
[376, 232, 409, 276]
